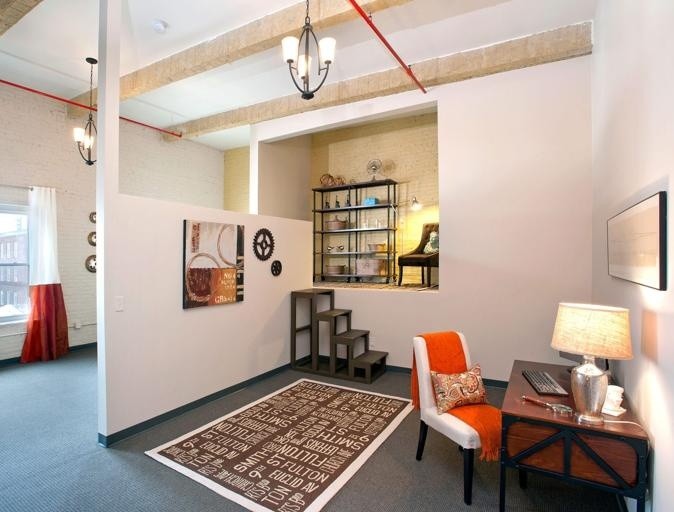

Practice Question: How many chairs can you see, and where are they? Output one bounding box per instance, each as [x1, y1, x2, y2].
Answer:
[398, 222, 439, 288]
[413, 331, 529, 505]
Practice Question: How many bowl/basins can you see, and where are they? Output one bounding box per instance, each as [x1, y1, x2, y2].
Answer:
[326, 264, 345, 274]
[366, 243, 385, 250]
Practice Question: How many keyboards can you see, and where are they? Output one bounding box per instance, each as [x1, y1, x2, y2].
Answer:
[521, 369, 570, 397]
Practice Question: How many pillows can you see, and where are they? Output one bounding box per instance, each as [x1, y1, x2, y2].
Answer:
[429, 362, 491, 415]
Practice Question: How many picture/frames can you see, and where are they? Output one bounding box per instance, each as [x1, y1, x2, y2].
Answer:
[607, 190, 667, 290]
[183, 219, 244, 310]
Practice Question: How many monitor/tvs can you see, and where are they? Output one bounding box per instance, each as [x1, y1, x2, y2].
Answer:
[558, 351, 610, 372]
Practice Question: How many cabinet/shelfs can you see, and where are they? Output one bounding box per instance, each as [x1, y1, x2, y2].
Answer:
[311, 179, 398, 285]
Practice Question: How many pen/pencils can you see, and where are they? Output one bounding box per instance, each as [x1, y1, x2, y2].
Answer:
[522, 396, 551, 407]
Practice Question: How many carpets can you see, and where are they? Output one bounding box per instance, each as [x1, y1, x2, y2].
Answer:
[143, 377, 416, 512]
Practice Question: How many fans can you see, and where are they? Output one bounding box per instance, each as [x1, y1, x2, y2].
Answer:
[365, 159, 383, 181]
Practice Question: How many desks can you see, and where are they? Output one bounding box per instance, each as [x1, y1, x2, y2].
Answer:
[498, 359, 650, 512]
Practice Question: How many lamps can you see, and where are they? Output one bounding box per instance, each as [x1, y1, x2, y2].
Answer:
[550, 301, 634, 427]
[280, 1, 337, 100]
[72, 58, 98, 166]
